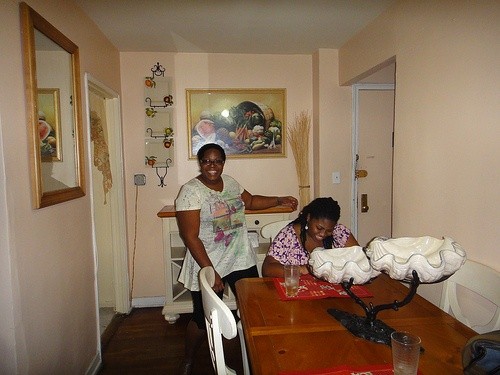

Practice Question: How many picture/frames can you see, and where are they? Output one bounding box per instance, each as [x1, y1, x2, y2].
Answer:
[36, 88, 64, 163]
[184, 88, 289, 161]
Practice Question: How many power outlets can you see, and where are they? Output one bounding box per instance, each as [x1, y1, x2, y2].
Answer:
[332, 171, 341, 184]
[134, 174, 146, 186]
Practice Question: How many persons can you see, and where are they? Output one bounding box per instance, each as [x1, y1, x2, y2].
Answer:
[262, 197, 360, 277]
[175, 143, 298, 375]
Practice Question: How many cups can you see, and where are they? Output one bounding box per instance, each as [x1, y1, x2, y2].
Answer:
[390, 331, 422, 375]
[284, 265, 300, 296]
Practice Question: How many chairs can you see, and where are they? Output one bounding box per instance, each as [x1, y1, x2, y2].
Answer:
[439, 259, 500, 334]
[199, 266, 237, 375]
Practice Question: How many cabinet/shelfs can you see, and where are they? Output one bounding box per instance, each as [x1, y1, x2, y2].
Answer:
[157, 205, 295, 324]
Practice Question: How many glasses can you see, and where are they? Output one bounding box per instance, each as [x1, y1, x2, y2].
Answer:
[200, 159, 224, 166]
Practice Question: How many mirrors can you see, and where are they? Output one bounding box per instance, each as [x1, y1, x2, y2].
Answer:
[18, 2, 86, 209]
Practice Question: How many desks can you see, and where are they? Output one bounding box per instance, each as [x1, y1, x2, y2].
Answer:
[234, 273, 500, 375]
